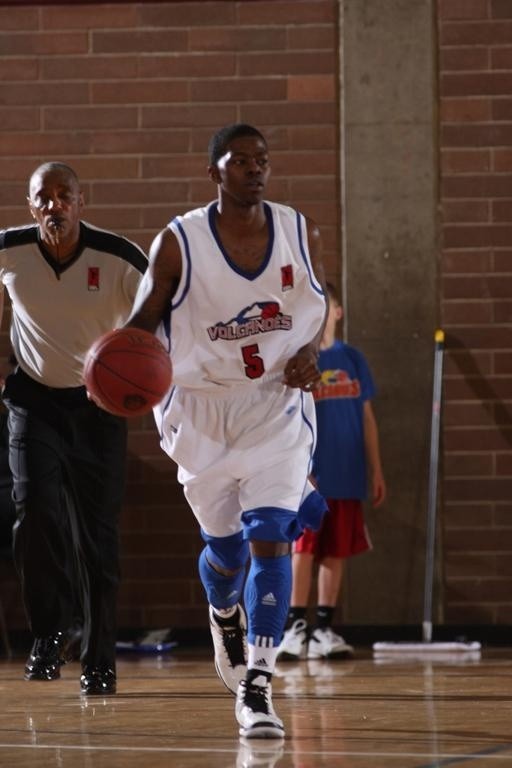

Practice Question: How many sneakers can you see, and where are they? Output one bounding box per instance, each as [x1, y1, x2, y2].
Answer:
[233, 662, 286, 739]
[308, 626, 355, 660]
[277, 627, 308, 663]
[207, 601, 249, 695]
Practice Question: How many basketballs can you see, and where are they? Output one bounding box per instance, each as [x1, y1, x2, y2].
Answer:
[83, 326, 172, 418]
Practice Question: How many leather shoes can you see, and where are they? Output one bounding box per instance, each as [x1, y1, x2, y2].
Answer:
[79, 664, 119, 695]
[23, 624, 74, 682]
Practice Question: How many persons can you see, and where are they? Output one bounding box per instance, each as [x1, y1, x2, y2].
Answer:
[0, 161, 149, 695]
[86, 123, 329, 739]
[275, 280, 386, 663]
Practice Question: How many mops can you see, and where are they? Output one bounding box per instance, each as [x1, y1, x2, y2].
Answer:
[371, 328, 484, 655]
